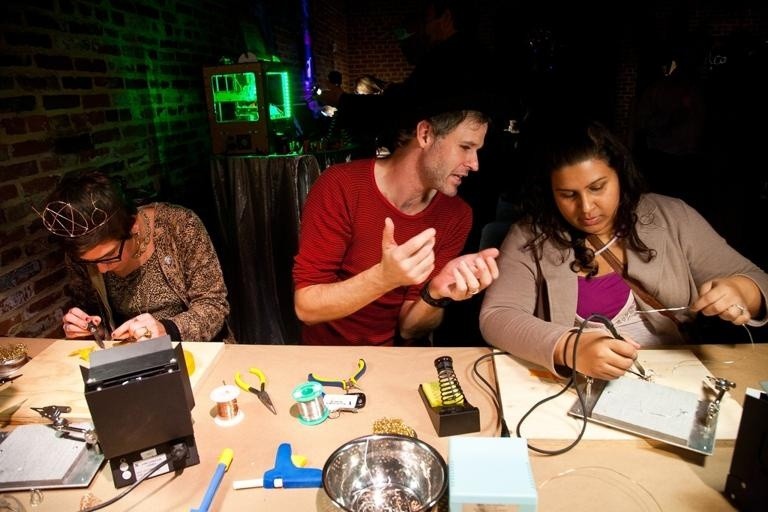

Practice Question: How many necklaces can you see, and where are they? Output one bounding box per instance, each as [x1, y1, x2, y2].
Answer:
[132, 209, 152, 259]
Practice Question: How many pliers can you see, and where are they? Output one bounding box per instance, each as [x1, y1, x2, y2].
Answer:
[307, 358, 367, 394]
[235, 367, 277, 416]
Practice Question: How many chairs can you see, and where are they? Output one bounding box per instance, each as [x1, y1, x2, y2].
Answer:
[211, 152, 323, 345]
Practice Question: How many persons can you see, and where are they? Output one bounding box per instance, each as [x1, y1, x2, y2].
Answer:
[42, 169, 238, 347]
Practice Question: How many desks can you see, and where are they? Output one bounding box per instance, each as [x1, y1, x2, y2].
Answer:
[1, 332, 766, 512]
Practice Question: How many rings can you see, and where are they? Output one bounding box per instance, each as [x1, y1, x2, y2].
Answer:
[62, 323, 67, 329]
[145, 330, 152, 338]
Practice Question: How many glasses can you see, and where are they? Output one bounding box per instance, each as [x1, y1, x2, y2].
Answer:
[76, 239, 124, 266]
